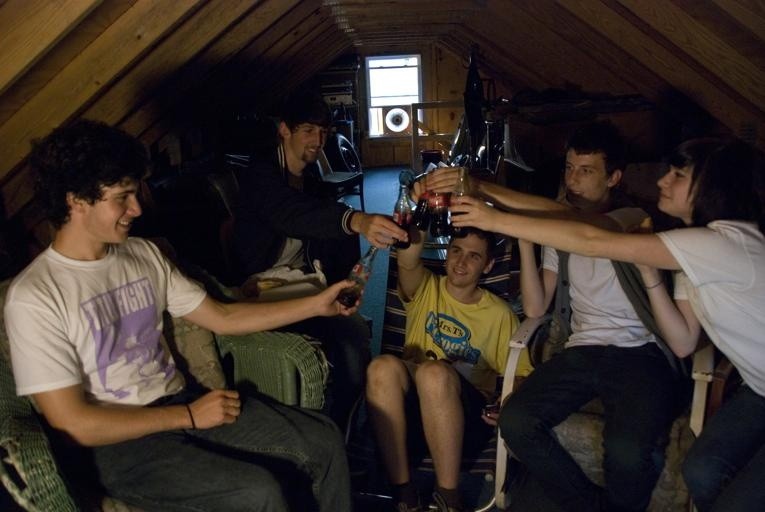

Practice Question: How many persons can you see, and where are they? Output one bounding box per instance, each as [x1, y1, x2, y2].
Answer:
[446, 134, 764, 512]
[0, 118, 362, 511]
[362, 162, 537, 511]
[408, 128, 691, 510]
[233, 85, 410, 426]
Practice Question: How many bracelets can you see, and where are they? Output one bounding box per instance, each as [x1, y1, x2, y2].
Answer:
[640, 279, 666, 292]
[186, 404, 195, 429]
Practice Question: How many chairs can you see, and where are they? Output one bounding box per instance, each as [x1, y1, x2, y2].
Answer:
[485, 220, 715, 511]
[2, 300, 331, 507]
[479, 159, 540, 211]
[375, 254, 523, 463]
[307, 148, 370, 217]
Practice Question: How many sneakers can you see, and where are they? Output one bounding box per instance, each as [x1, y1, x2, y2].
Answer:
[389, 488, 421, 512]
[426, 492, 455, 512]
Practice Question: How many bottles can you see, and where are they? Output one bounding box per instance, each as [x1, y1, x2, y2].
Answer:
[389, 184, 413, 248]
[430, 192, 448, 238]
[451, 167, 472, 236]
[413, 191, 431, 232]
[337, 244, 379, 307]
